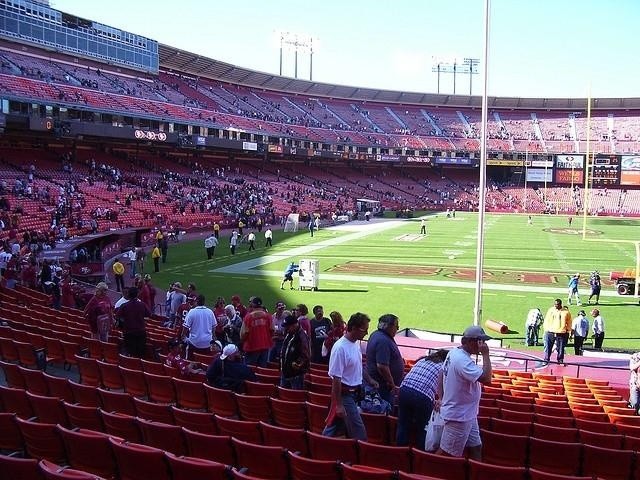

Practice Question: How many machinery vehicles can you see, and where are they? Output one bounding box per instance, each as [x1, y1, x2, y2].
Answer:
[396, 206, 414, 218]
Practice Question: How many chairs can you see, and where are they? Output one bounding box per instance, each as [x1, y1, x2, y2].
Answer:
[1, 279, 640, 478]
[1, 50, 325, 279]
[325, 90, 640, 219]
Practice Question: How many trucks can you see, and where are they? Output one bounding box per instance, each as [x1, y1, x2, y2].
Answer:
[614, 277, 640, 295]
[357, 197, 386, 217]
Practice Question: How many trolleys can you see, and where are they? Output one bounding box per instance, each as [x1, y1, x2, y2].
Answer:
[297, 259, 320, 292]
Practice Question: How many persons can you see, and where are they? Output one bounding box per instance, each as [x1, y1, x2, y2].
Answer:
[1, 45, 639, 456]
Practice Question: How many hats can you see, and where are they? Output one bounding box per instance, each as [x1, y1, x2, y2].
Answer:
[220, 343, 241, 361]
[462, 326, 493, 341]
[173, 281, 286, 317]
[281, 314, 297, 328]
[209, 339, 223, 351]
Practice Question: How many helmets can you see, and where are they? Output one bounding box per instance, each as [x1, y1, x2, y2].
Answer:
[577, 310, 586, 317]
[590, 309, 599, 318]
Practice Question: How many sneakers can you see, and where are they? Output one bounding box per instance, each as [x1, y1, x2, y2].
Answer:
[542, 359, 549, 366]
[557, 359, 566, 366]
